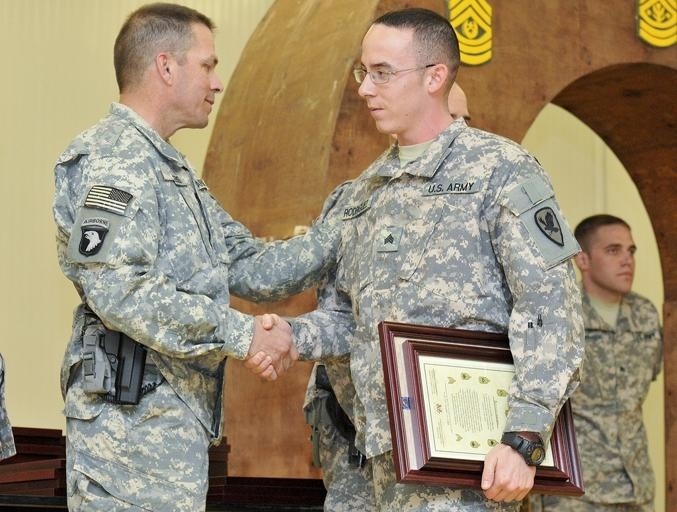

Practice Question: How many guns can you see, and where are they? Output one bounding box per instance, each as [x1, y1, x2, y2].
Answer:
[315, 365, 366, 468]
[80, 303, 147, 405]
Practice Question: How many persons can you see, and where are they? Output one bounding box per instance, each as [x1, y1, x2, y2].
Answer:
[302, 83, 476, 509]
[240, 5, 589, 512]
[48, 3, 342, 512]
[538, 213, 668, 512]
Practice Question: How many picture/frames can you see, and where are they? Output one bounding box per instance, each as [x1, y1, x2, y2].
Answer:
[377, 321, 585, 498]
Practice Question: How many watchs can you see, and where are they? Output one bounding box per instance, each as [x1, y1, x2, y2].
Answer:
[498, 431, 550, 470]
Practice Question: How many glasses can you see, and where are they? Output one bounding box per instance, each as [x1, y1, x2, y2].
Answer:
[353, 64, 435, 84]
